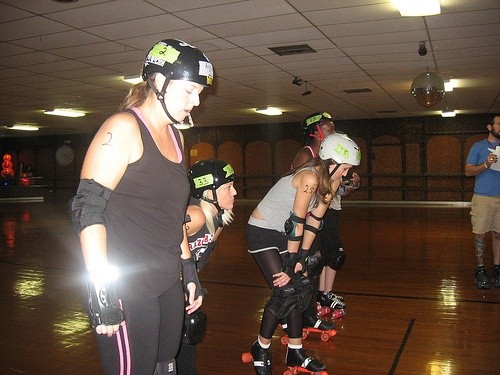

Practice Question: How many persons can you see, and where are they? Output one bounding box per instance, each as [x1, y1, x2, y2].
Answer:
[68, 38, 214, 375]
[176, 161, 238, 375]
[242, 112, 362, 375]
[465, 115, 500, 289]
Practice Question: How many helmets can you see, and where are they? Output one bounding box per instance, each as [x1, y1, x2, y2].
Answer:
[187, 158, 237, 199]
[318, 133, 361, 166]
[303, 111, 335, 135]
[142, 39, 214, 88]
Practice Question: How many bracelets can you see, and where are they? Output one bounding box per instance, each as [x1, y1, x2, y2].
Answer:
[485, 161, 489, 168]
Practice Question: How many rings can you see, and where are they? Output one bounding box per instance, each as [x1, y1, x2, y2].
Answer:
[490, 158, 493, 161]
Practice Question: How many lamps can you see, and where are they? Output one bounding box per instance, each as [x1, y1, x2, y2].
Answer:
[418, 41, 427, 56]
[292, 77, 302, 86]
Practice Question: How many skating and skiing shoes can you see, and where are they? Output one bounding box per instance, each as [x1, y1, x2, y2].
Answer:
[278, 317, 307, 344]
[490, 266, 500, 287]
[316, 290, 344, 302]
[316, 293, 346, 319]
[472, 270, 490, 289]
[241, 336, 273, 375]
[302, 308, 336, 342]
[283, 347, 329, 375]
